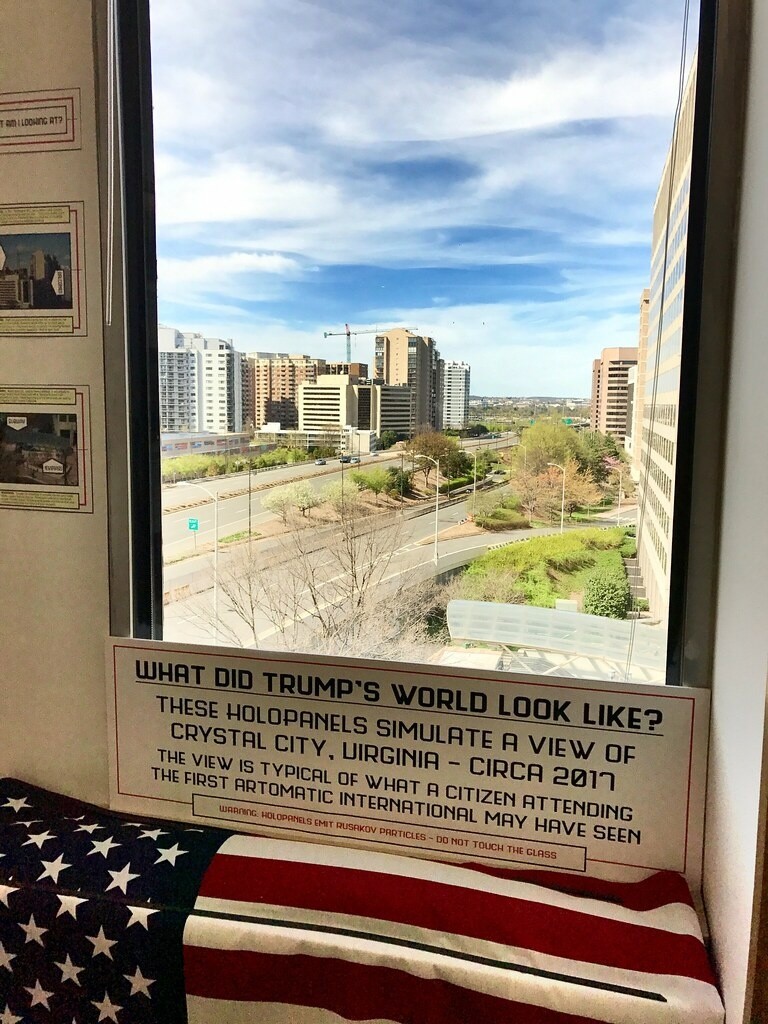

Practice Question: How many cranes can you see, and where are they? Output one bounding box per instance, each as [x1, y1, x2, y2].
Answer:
[324, 323, 419, 363]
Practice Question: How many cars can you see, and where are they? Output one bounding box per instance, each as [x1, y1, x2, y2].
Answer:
[340, 455, 352, 463]
[350, 457, 361, 464]
[491, 433, 501, 437]
[370, 451, 378, 456]
[314, 459, 326, 465]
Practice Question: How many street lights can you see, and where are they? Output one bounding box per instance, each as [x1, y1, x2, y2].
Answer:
[235, 459, 252, 561]
[335, 451, 343, 526]
[510, 443, 527, 476]
[606, 465, 621, 526]
[354, 432, 360, 470]
[459, 451, 477, 519]
[414, 455, 439, 566]
[397, 453, 403, 515]
[176, 482, 219, 645]
[547, 463, 565, 534]
